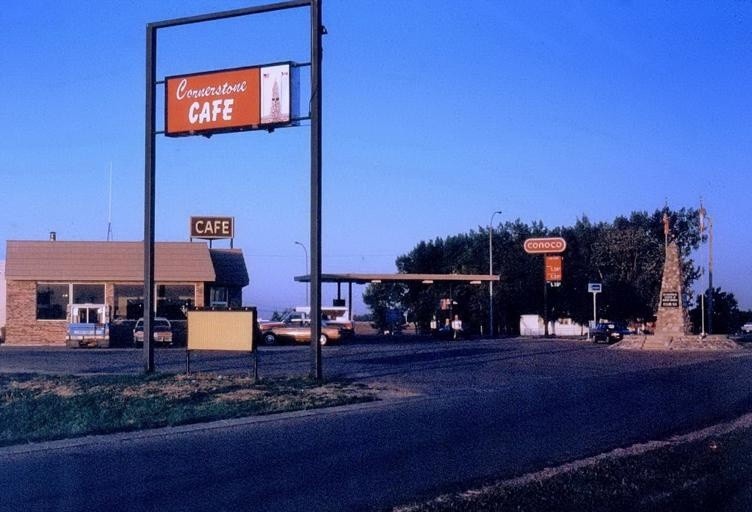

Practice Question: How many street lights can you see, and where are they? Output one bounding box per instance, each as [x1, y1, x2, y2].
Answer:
[295, 240, 309, 307]
[489, 209, 504, 340]
[699, 213, 714, 336]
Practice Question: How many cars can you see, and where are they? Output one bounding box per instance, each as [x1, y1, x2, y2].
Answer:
[259, 306, 355, 346]
[437, 324, 477, 339]
[726, 331, 752, 346]
[589, 322, 633, 346]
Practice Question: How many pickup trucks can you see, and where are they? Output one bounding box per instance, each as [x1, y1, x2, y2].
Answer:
[132, 316, 177, 347]
